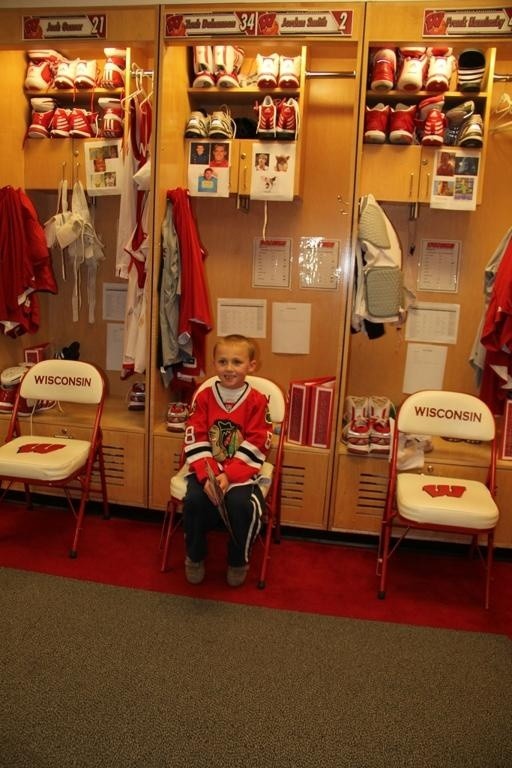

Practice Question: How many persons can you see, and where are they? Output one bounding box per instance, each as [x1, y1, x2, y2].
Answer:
[93, 160, 106, 171]
[191, 144, 209, 164]
[257, 153, 269, 171]
[436, 181, 450, 196]
[111, 147, 116, 158]
[94, 175, 103, 186]
[439, 152, 454, 177]
[183, 334, 276, 588]
[199, 168, 216, 192]
[211, 144, 228, 166]
[107, 173, 114, 187]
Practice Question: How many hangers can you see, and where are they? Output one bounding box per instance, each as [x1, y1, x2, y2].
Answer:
[121, 62, 154, 115]
[489, 111, 512, 131]
[491, 74, 512, 117]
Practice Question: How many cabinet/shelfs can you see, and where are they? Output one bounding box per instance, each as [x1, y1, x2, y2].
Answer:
[149, 2, 367, 530]
[330, 1, 512, 549]
[0, 0, 157, 509]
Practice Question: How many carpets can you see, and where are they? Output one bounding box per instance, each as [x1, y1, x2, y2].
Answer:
[0, 498, 511, 635]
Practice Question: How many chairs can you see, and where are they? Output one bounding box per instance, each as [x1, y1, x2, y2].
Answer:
[160, 375, 287, 589]
[377, 389, 500, 610]
[0, 358, 109, 557]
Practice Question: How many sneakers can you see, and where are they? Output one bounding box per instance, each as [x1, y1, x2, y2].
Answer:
[454, 114, 484, 147]
[389, 102, 417, 144]
[26, 60, 57, 89]
[103, 46, 127, 67]
[209, 112, 237, 139]
[99, 97, 124, 114]
[365, 102, 390, 144]
[101, 115, 123, 138]
[52, 108, 71, 136]
[193, 45, 213, 69]
[426, 46, 453, 57]
[1, 365, 33, 384]
[278, 55, 302, 88]
[457, 48, 485, 90]
[253, 95, 277, 138]
[460, 82, 480, 92]
[125, 381, 145, 410]
[168, 401, 187, 432]
[1, 383, 20, 411]
[102, 62, 125, 87]
[418, 95, 447, 119]
[415, 109, 446, 145]
[399, 55, 429, 89]
[226, 564, 251, 587]
[369, 416, 392, 455]
[398, 47, 427, 70]
[276, 97, 299, 134]
[186, 110, 208, 138]
[75, 59, 99, 88]
[55, 62, 74, 89]
[371, 56, 394, 90]
[18, 384, 65, 435]
[214, 46, 247, 73]
[447, 101, 475, 127]
[216, 70, 240, 87]
[426, 57, 451, 90]
[373, 47, 396, 67]
[29, 109, 54, 138]
[368, 395, 397, 418]
[186, 556, 206, 583]
[32, 96, 57, 111]
[345, 395, 369, 420]
[256, 52, 279, 85]
[72, 107, 96, 137]
[341, 417, 370, 453]
[195, 72, 214, 89]
[26, 48, 67, 63]
